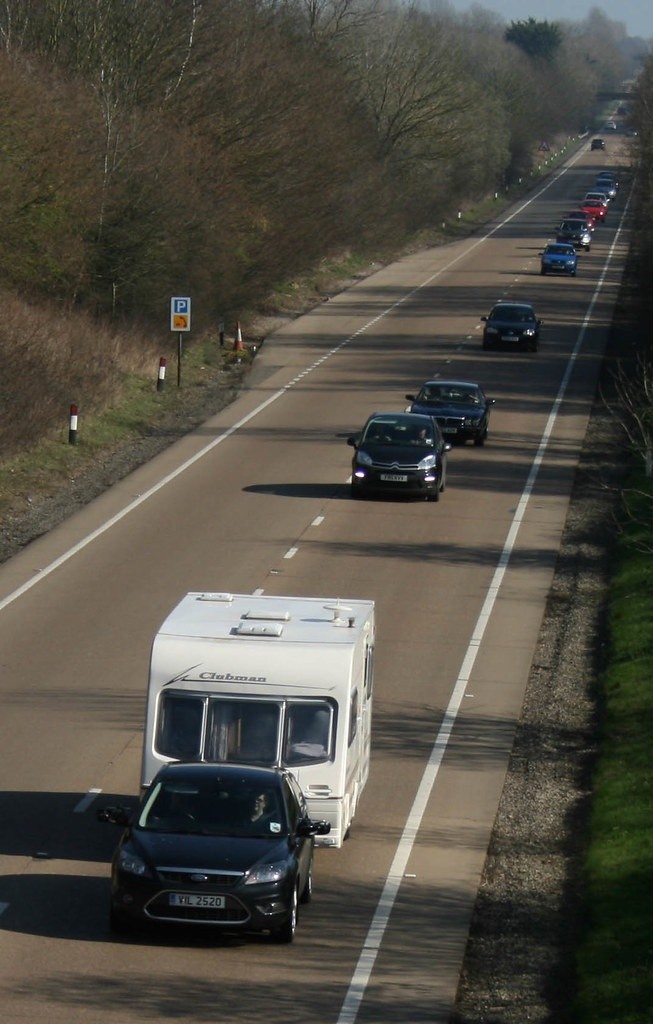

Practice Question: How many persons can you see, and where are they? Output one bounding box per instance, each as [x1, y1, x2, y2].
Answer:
[414, 427, 430, 442]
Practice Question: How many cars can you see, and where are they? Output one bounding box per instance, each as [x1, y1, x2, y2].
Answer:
[604, 121, 617, 130]
[346, 412, 452, 502]
[597, 171, 620, 190]
[563, 209, 596, 235]
[593, 178, 619, 201]
[554, 217, 594, 251]
[578, 199, 608, 223]
[480, 303, 544, 352]
[405, 378, 497, 448]
[102, 759, 331, 945]
[591, 138, 606, 151]
[584, 191, 611, 209]
[539, 243, 581, 277]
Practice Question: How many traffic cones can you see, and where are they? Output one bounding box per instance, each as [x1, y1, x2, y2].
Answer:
[232, 320, 245, 352]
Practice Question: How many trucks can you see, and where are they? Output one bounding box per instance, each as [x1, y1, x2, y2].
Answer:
[139, 591, 377, 849]
